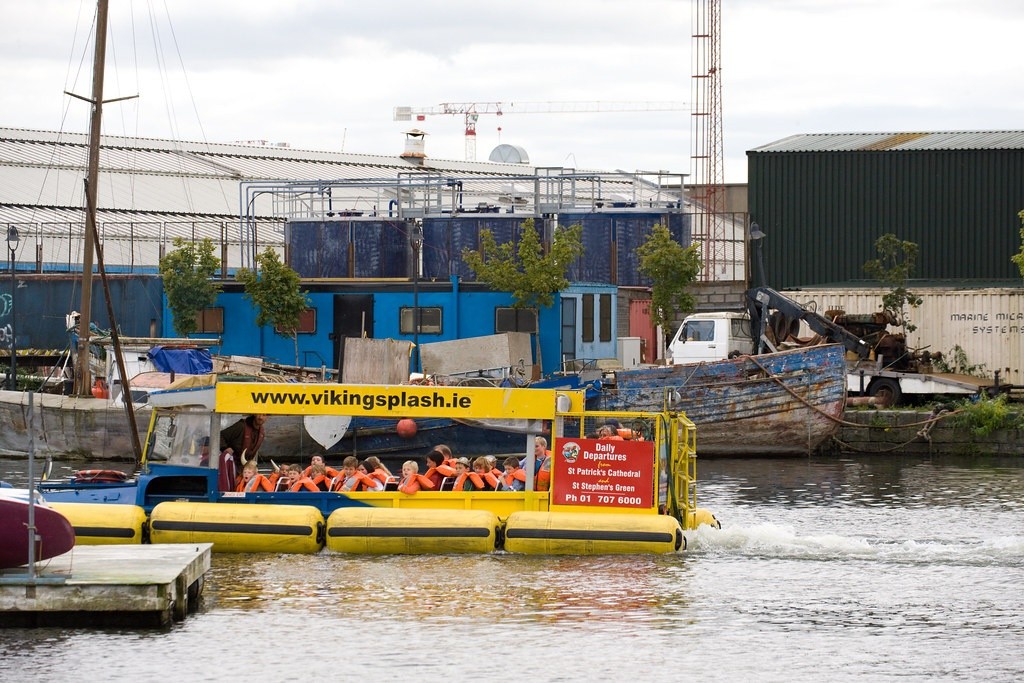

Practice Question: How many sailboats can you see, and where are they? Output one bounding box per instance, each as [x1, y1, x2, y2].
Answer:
[0, 0, 848, 457]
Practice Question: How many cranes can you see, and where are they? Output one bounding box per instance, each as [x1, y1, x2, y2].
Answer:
[390, 102, 722, 163]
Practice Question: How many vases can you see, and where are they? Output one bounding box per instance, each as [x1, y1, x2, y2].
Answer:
[91, 376, 109, 399]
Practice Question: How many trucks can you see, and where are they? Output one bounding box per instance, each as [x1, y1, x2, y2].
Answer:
[653, 311, 1002, 410]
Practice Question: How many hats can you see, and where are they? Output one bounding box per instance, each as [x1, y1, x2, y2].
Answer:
[195, 436, 210, 446]
[455, 456, 473, 467]
[484, 454, 498, 463]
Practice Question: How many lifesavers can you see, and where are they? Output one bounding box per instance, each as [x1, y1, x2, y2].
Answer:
[867, 378, 901, 405]
[75, 469, 126, 483]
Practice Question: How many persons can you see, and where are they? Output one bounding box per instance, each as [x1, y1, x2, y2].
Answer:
[236, 463, 274, 494]
[268, 445, 525, 492]
[219, 413, 268, 464]
[581, 425, 649, 442]
[519, 436, 552, 491]
[196, 436, 223, 469]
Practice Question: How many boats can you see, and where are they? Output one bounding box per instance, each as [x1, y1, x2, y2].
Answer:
[33, 374, 720, 556]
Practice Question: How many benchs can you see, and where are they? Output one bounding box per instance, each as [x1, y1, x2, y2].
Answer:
[495, 481, 504, 492]
[382, 476, 401, 491]
[329, 477, 346, 491]
[440, 477, 456, 491]
[274, 476, 290, 493]
